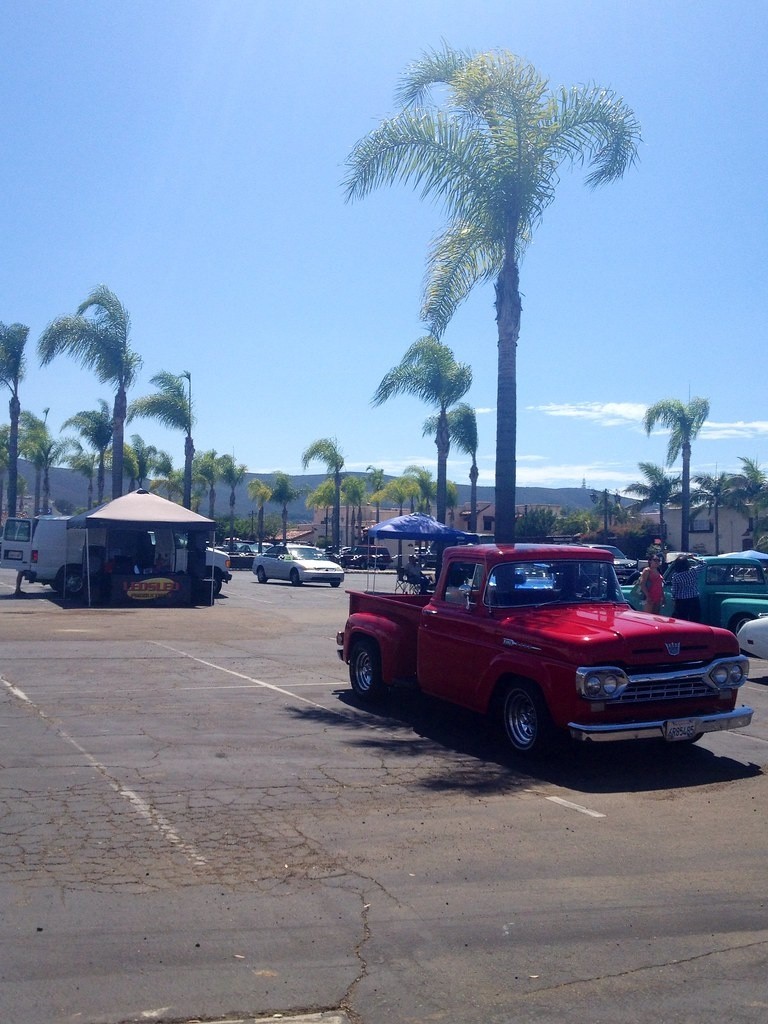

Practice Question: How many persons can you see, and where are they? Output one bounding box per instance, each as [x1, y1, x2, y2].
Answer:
[640, 554, 665, 615]
[402, 555, 430, 594]
[671, 554, 708, 622]
[15, 524, 35, 595]
[656, 549, 670, 576]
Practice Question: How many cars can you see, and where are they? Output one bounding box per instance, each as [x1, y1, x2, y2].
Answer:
[214, 538, 275, 569]
[341, 546, 393, 570]
[621, 557, 768, 657]
[584, 545, 639, 585]
[252, 544, 345, 587]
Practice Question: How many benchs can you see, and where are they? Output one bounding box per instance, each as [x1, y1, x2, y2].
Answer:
[447, 588, 558, 607]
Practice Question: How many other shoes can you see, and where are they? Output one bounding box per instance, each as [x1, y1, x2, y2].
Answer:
[15, 591, 25, 597]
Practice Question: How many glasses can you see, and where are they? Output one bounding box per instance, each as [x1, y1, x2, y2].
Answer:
[651, 559, 661, 562]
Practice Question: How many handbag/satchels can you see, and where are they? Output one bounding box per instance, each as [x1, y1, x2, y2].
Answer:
[630, 579, 652, 600]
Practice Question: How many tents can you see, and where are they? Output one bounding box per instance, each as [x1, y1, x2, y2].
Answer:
[64, 488, 216, 608]
[718, 550, 768, 581]
[367, 513, 481, 592]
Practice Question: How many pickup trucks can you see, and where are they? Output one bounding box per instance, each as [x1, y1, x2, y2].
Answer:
[336, 545, 754, 761]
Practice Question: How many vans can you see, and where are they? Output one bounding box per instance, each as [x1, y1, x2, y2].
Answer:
[0, 515, 233, 597]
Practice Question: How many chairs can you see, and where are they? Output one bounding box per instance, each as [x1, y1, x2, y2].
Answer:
[395, 567, 421, 594]
